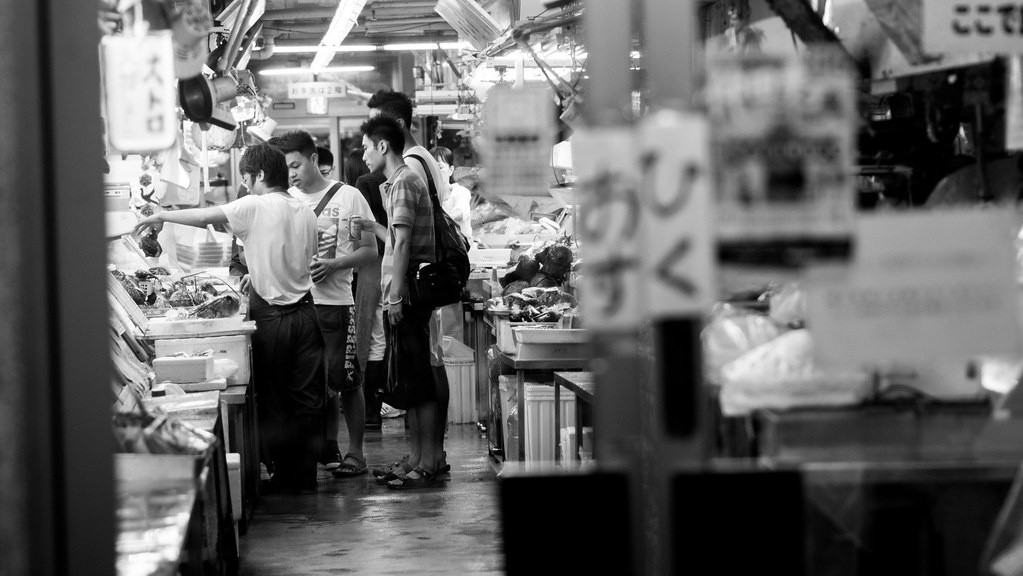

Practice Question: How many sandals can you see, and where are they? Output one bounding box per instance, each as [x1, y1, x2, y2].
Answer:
[376, 464, 412, 485]
[387, 466, 440, 487]
[331, 454, 369, 476]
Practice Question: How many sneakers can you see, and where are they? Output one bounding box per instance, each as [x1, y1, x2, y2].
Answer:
[364, 415, 382, 428]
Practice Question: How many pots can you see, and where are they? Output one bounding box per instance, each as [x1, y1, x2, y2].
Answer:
[180, 72, 214, 123]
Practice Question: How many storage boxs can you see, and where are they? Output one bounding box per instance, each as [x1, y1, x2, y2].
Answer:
[155, 334, 251, 387]
[441, 356, 480, 424]
[501, 375, 580, 465]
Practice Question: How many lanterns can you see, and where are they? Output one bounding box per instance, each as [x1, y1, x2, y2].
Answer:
[191, 107, 238, 152]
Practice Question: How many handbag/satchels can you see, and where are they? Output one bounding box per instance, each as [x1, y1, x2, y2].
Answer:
[431, 203, 470, 282]
[406, 260, 463, 308]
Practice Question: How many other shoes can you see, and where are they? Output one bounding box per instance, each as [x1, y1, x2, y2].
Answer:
[322, 453, 343, 468]
[372, 456, 410, 477]
[434, 461, 452, 480]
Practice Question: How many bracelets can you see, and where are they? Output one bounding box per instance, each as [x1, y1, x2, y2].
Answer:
[387, 296, 403, 305]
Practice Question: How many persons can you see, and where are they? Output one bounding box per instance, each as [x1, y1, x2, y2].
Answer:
[351, 111, 453, 489]
[236, 137, 288, 297]
[351, 169, 409, 419]
[427, 146, 478, 260]
[261, 129, 379, 477]
[363, 88, 449, 475]
[342, 150, 369, 192]
[134, 143, 328, 497]
[295, 146, 351, 469]
